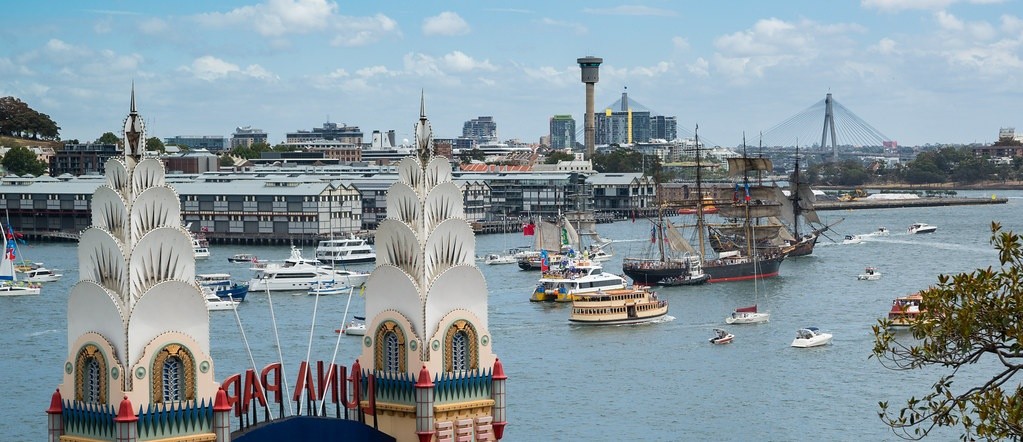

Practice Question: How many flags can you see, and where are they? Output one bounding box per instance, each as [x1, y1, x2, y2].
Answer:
[523, 221, 536, 235]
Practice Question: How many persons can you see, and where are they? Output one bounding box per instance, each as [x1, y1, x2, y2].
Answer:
[543, 271, 584, 279]
[893, 299, 918, 306]
[8, 280, 40, 288]
[715, 252, 778, 265]
[624, 261, 685, 268]
[865, 266, 874, 275]
[663, 274, 692, 282]
[715, 330, 726, 339]
[731, 311, 736, 318]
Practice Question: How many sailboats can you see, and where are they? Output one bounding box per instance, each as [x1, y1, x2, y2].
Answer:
[308, 189, 355, 296]
[0, 209, 40, 296]
[725, 223, 771, 323]
[623, 125, 845, 281]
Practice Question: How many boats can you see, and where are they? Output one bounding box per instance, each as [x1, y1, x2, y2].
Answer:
[874, 227, 889, 237]
[475, 201, 668, 324]
[656, 273, 711, 286]
[196, 274, 249, 311]
[889, 292, 948, 331]
[228, 254, 253, 262]
[858, 272, 881, 281]
[708, 329, 734, 345]
[843, 235, 861, 244]
[193, 240, 209, 259]
[14, 260, 62, 282]
[316, 233, 376, 264]
[908, 223, 937, 234]
[790, 327, 833, 348]
[346, 316, 365, 335]
[249, 237, 370, 291]
